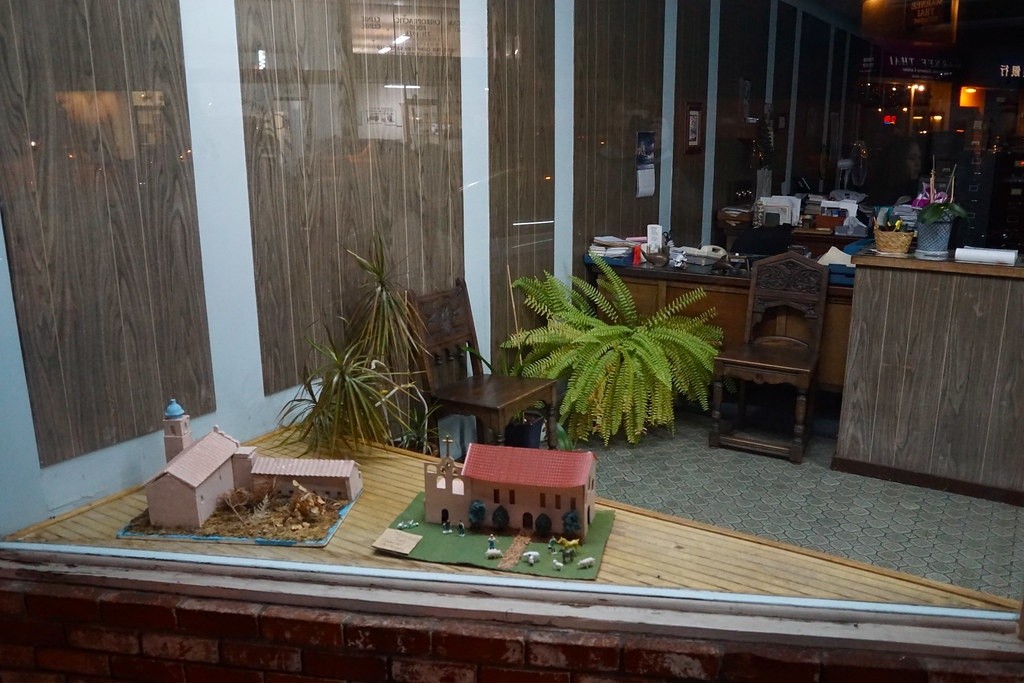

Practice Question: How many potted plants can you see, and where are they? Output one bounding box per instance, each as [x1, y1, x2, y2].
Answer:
[457, 332, 546, 447]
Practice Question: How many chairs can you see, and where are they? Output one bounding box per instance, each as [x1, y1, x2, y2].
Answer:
[707, 250, 830, 466]
[403, 277, 560, 458]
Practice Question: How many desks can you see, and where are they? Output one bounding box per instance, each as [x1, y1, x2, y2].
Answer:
[723, 229, 861, 259]
[588, 263, 854, 386]
[0, 422, 1023, 613]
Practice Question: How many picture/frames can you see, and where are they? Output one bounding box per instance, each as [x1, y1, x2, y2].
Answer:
[775, 113, 789, 129]
[684, 101, 705, 156]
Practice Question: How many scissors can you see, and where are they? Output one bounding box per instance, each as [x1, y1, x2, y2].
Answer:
[662, 230, 673, 241]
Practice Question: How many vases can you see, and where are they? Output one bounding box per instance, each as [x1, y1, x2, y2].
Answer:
[916, 209, 956, 251]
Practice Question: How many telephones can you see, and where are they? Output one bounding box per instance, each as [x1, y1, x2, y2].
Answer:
[673, 244, 726, 266]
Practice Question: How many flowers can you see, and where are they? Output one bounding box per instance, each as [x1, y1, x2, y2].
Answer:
[912, 182, 971, 225]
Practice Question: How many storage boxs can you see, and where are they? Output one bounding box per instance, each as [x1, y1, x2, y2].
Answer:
[835, 224, 871, 238]
[718, 220, 753, 230]
[816, 215, 845, 230]
[584, 253, 633, 267]
[717, 210, 753, 222]
